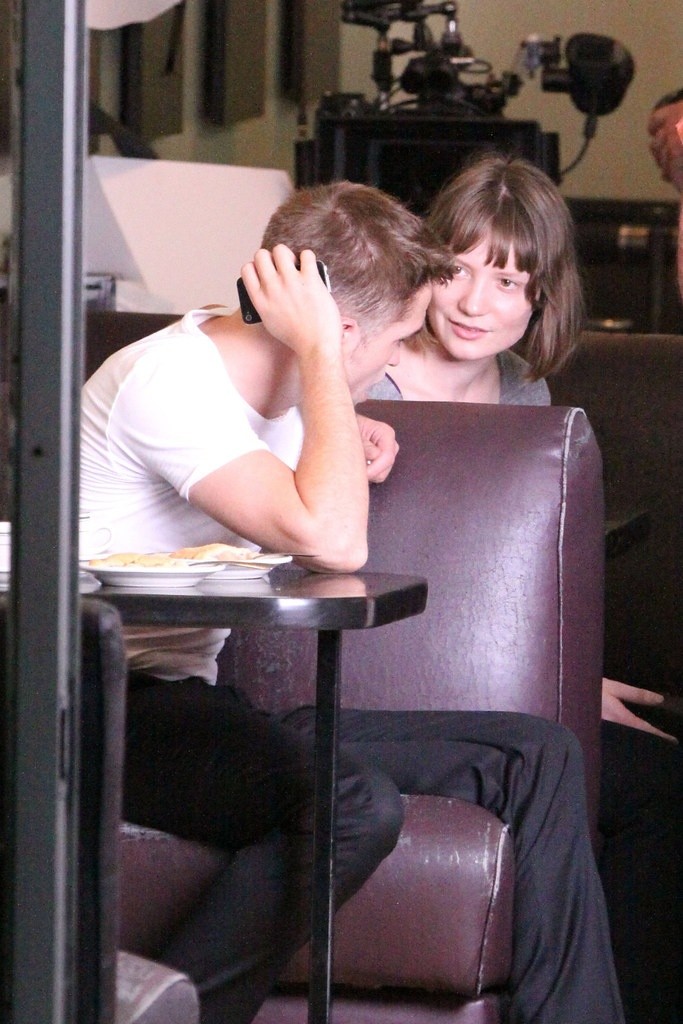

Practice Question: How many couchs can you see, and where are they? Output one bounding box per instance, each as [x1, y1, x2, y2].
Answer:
[0, 308, 683, 1024]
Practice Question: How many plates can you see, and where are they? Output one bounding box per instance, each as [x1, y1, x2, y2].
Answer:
[168, 552, 293, 580]
[78, 559, 225, 587]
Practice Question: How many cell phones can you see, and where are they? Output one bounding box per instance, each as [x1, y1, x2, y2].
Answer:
[237, 260, 331, 324]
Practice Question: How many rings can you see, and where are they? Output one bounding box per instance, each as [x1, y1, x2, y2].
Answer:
[646, 138, 665, 152]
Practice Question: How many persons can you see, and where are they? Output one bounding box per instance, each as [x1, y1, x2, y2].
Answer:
[646, 88, 683, 193]
[77, 151, 683, 1024]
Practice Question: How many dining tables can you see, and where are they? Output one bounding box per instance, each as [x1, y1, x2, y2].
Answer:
[0, 569, 429, 1024]
